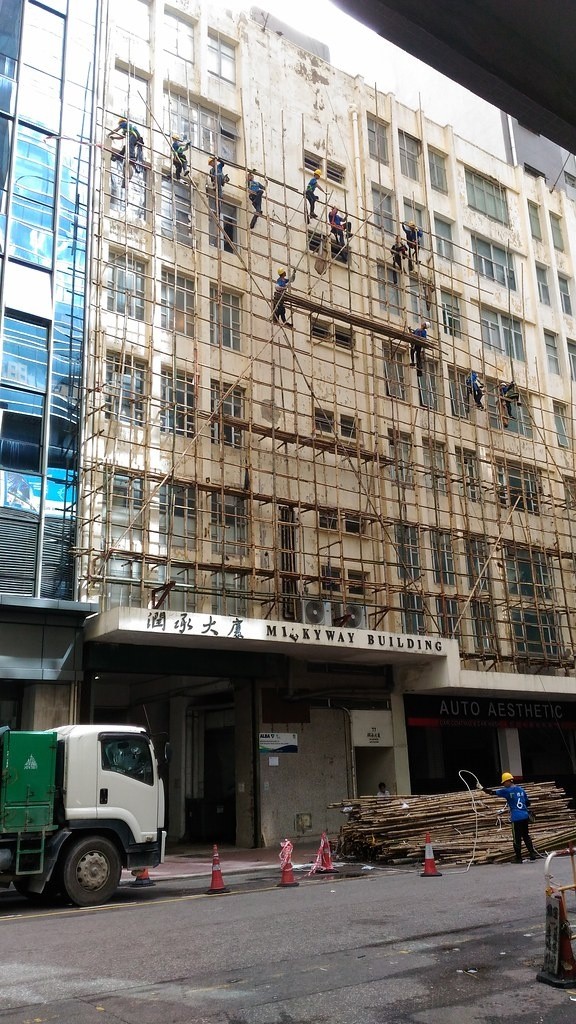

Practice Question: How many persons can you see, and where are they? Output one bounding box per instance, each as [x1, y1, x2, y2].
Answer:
[402, 221, 423, 264]
[272, 268, 293, 327]
[328, 206, 354, 263]
[390, 235, 413, 272]
[408, 321, 431, 369]
[171, 134, 191, 180]
[466, 371, 484, 411]
[476, 772, 537, 864]
[245, 173, 268, 214]
[207, 156, 224, 198]
[377, 782, 391, 795]
[306, 169, 327, 218]
[108, 118, 140, 159]
[500, 381, 522, 420]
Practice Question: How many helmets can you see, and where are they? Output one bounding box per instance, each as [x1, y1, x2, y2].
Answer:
[501, 772, 513, 785]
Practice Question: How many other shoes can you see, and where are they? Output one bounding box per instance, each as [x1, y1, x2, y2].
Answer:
[512, 858, 522, 864]
[530, 857, 538, 862]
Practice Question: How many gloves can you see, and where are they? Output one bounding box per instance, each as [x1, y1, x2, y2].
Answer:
[476, 782, 484, 790]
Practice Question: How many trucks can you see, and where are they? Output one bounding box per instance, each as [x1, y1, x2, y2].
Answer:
[0, 723, 170, 905]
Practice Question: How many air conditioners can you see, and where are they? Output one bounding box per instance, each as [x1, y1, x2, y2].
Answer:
[333, 603, 366, 630]
[295, 600, 333, 627]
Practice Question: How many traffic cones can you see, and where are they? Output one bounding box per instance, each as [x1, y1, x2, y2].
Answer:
[536, 895, 576, 989]
[204, 844, 231, 894]
[420, 832, 443, 877]
[131, 865, 156, 887]
[276, 839, 300, 887]
[315, 837, 339, 873]
